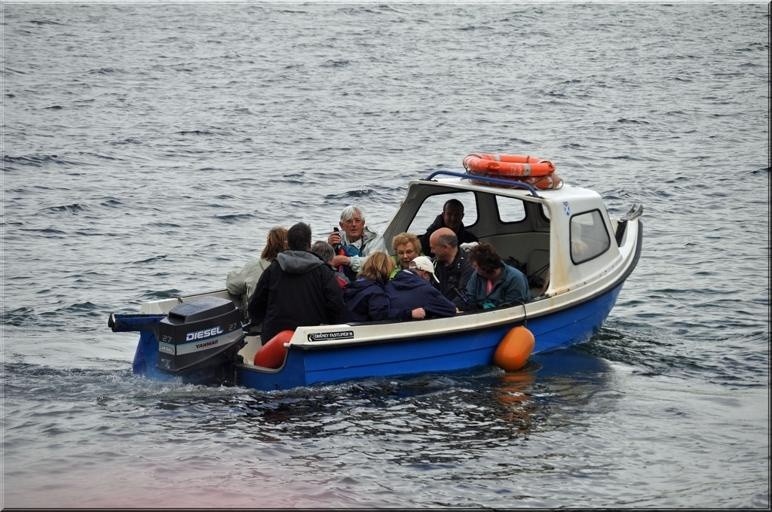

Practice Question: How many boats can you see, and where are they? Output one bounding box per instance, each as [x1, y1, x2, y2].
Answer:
[105, 152, 644, 392]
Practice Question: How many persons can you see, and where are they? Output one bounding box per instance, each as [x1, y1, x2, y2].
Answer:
[325, 205, 389, 285]
[338, 251, 427, 326]
[310, 239, 350, 288]
[223, 228, 290, 321]
[418, 198, 478, 261]
[379, 254, 459, 319]
[452, 242, 530, 314]
[426, 226, 481, 302]
[248, 222, 345, 343]
[379, 230, 423, 283]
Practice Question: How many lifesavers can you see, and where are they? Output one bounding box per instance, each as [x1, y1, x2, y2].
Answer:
[469, 172, 562, 192]
[464, 152, 555, 176]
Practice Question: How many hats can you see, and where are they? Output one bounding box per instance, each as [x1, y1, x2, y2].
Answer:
[410, 255, 440, 283]
